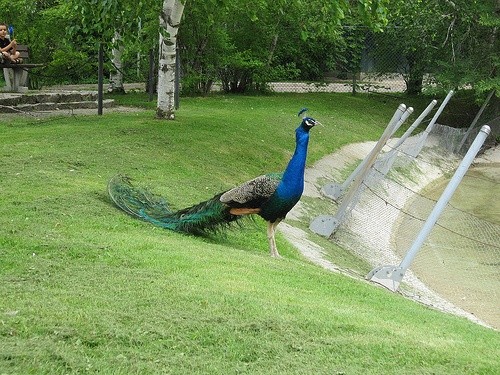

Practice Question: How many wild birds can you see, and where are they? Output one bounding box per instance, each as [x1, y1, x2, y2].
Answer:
[106, 107, 324, 261]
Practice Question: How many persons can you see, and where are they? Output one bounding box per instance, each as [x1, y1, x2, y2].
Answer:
[0, 23, 21, 64]
